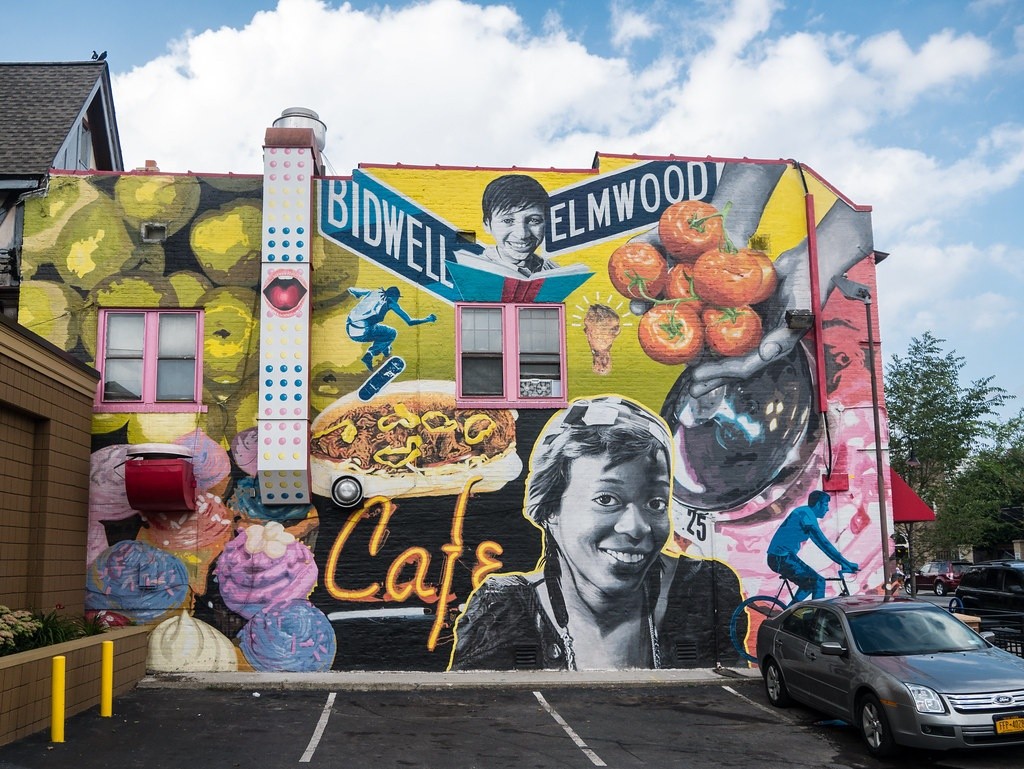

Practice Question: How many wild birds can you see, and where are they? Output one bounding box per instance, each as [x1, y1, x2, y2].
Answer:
[91, 51, 107, 61]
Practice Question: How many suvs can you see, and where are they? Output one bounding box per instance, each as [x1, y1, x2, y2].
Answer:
[904, 559, 977, 596]
[954, 559, 1024, 624]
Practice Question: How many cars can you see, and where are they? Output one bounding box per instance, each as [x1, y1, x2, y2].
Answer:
[756, 594, 1024, 757]
